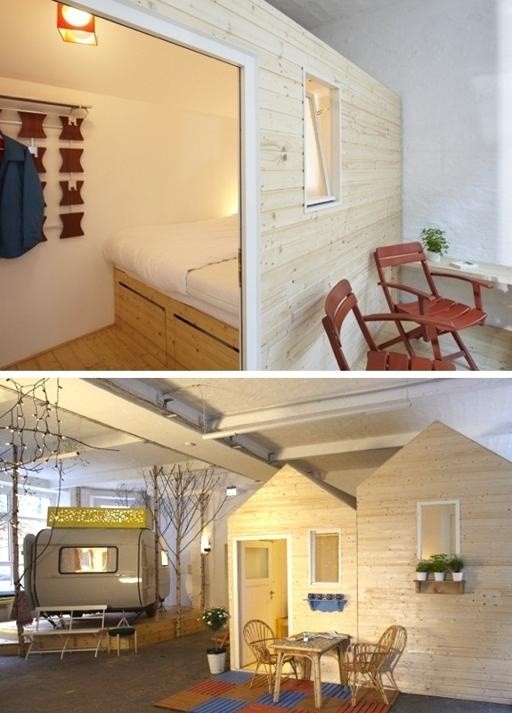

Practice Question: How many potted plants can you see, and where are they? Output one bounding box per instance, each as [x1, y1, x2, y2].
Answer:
[419, 226, 449, 261]
[196, 607, 231, 674]
[415, 553, 464, 581]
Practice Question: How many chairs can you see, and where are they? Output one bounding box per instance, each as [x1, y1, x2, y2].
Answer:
[320, 241, 492, 369]
[242, 618, 397, 706]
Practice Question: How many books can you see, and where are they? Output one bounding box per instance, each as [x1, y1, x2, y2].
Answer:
[450, 260, 477, 269]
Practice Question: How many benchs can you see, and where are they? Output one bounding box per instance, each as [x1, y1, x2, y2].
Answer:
[21, 604, 108, 660]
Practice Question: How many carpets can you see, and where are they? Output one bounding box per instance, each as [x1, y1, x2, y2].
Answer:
[153, 669, 400, 713]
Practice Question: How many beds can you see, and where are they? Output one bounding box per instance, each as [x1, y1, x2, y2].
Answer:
[104, 212, 240, 369]
